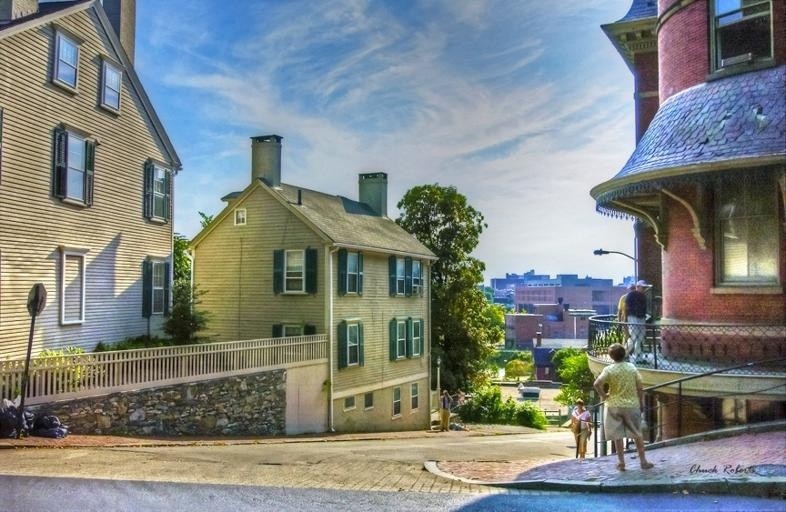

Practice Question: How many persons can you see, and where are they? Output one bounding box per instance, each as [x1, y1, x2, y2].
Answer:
[570, 398, 592, 458]
[617, 283, 636, 342]
[593, 341, 654, 471]
[625, 279, 653, 365]
[439, 390, 453, 431]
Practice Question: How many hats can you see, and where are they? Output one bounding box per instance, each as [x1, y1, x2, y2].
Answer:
[576, 398, 584, 404]
[637, 280, 649, 288]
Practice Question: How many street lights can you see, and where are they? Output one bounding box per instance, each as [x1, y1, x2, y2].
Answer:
[593, 237, 638, 289]
[435, 356, 441, 409]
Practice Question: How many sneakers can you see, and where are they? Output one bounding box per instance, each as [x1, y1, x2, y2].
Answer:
[641, 462, 654, 468]
[616, 464, 626, 471]
